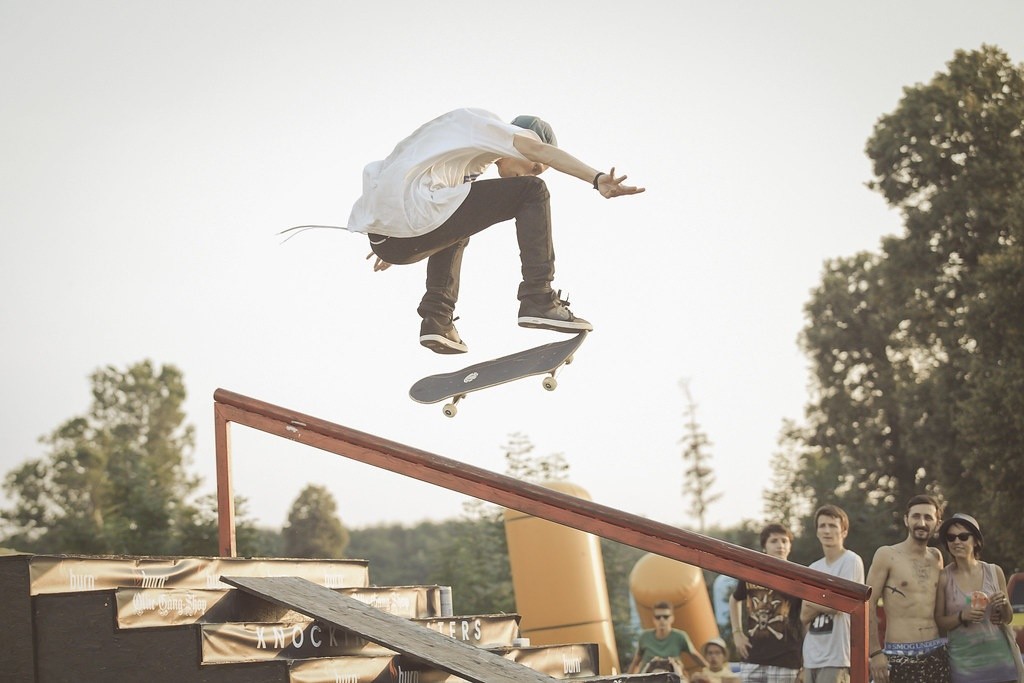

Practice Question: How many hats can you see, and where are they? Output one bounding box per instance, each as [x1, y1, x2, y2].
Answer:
[939, 513, 984, 554]
[701, 638, 730, 663]
[511, 115, 557, 147]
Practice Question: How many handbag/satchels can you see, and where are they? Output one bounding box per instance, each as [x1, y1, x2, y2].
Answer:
[990, 564, 1024, 683]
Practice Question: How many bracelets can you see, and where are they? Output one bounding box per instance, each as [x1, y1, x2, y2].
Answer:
[959, 610, 968, 627]
[870, 650, 883, 658]
[593, 171, 605, 190]
[732, 627, 742, 633]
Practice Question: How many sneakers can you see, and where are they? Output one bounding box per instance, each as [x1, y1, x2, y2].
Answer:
[419, 315, 468, 355]
[518, 289, 593, 333]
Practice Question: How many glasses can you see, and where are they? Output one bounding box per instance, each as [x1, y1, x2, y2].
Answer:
[945, 532, 974, 541]
[655, 615, 669, 619]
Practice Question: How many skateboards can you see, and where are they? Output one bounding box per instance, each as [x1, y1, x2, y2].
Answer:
[408, 329, 592, 405]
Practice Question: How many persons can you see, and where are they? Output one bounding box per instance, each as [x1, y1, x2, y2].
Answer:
[346, 107, 645, 355]
[627, 602, 710, 683]
[800, 504, 864, 683]
[729, 523, 803, 683]
[866, 495, 1003, 683]
[933, 513, 1024, 683]
[692, 638, 740, 683]
